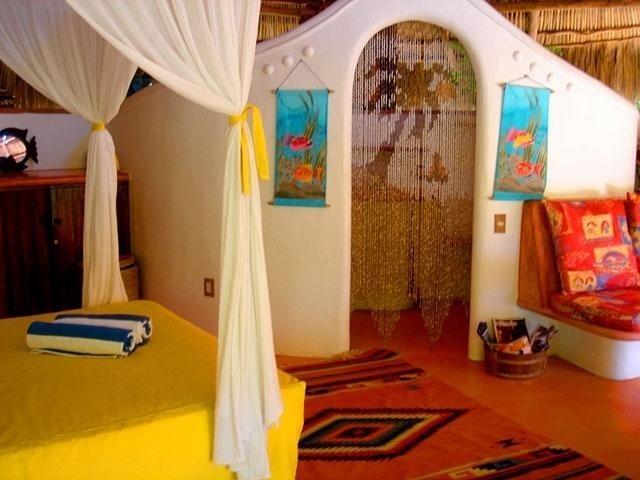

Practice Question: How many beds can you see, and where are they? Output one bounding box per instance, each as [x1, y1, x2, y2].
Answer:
[1, 299, 306, 480]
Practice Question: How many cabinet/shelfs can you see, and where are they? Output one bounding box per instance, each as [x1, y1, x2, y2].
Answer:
[0, 167, 130, 318]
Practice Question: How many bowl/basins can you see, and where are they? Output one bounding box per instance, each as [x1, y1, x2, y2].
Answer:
[485, 339, 550, 378]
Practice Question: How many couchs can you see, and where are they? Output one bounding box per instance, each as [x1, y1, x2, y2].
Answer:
[520, 192, 640, 380]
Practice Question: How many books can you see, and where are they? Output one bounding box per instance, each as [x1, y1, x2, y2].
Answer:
[476, 317, 558, 356]
